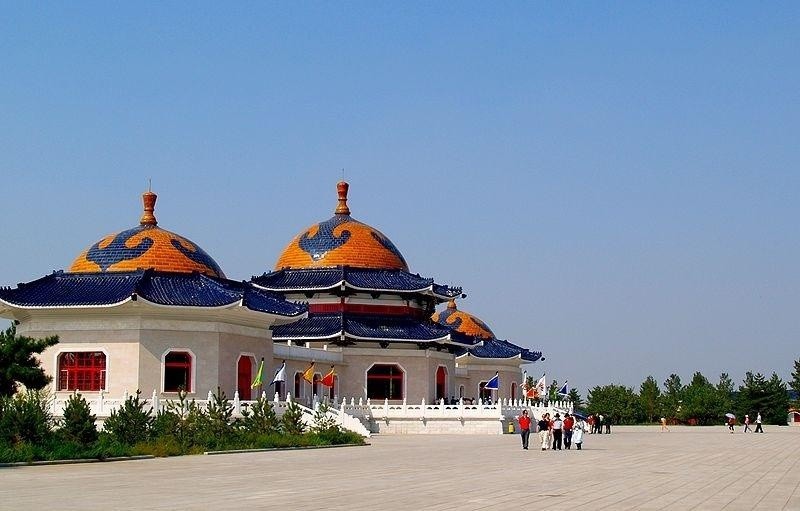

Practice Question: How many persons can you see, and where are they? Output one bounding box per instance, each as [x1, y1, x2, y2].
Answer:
[518, 409, 531, 450]
[661, 415, 670, 432]
[754, 412, 763, 433]
[537, 413, 611, 450]
[743, 415, 752, 433]
[435, 395, 475, 404]
[484, 398, 488, 405]
[488, 396, 493, 405]
[729, 419, 735, 433]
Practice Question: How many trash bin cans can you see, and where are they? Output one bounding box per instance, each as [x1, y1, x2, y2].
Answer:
[508, 422, 515, 433]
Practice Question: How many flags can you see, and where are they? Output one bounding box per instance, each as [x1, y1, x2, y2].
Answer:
[550, 386, 557, 399]
[519, 373, 526, 393]
[318, 367, 335, 388]
[522, 387, 533, 398]
[268, 362, 286, 385]
[483, 372, 499, 390]
[533, 375, 547, 395]
[300, 364, 315, 384]
[251, 360, 265, 389]
[557, 382, 569, 395]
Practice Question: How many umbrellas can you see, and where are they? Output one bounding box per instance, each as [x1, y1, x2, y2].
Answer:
[725, 413, 736, 419]
